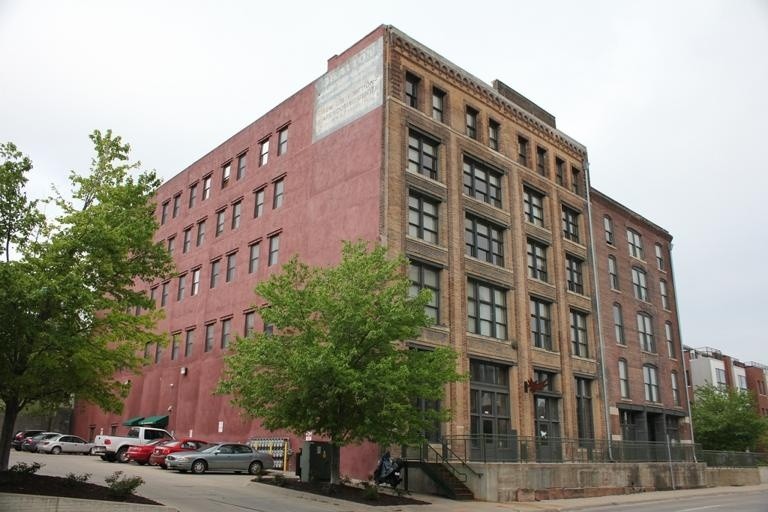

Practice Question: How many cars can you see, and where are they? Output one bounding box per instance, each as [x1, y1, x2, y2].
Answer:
[12, 425, 275, 477]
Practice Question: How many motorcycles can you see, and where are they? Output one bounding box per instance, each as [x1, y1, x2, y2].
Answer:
[374, 449, 405, 490]
